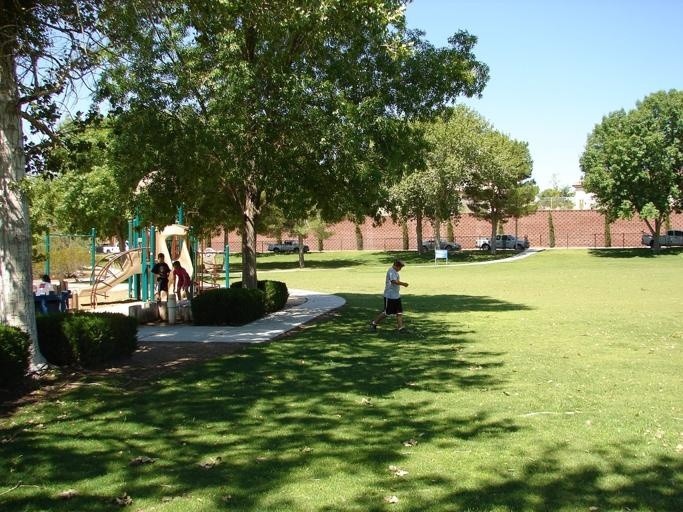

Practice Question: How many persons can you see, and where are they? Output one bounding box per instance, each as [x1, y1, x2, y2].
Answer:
[151, 253, 171, 302]
[370, 261, 409, 334]
[172, 261, 190, 302]
[40, 274, 54, 296]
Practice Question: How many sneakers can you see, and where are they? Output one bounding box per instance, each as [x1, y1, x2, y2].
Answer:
[399, 328, 409, 334]
[370, 320, 377, 333]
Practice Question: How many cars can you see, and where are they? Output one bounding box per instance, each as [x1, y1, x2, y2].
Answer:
[416, 239, 461, 254]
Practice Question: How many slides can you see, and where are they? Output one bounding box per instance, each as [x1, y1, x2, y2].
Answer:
[74, 251, 142, 298]
[157, 225, 194, 281]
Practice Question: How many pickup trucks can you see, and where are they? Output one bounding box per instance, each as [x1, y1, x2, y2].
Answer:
[640, 230, 683, 247]
[102, 242, 129, 254]
[267, 240, 308, 254]
[475, 234, 529, 251]
